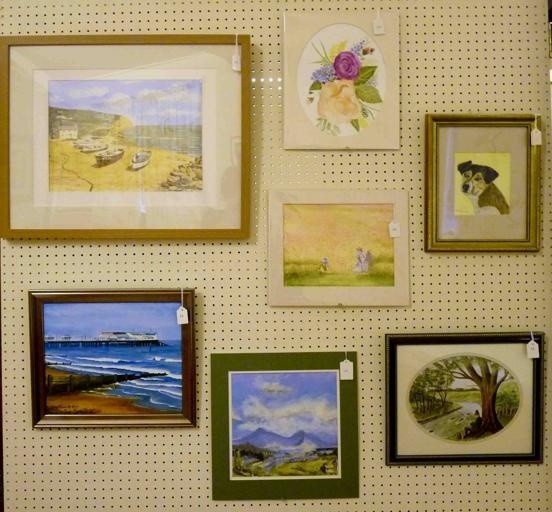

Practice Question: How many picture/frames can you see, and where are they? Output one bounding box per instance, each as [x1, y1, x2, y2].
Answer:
[28, 290, 198, 429]
[207, 350, 362, 499]
[265, 189, 408, 311]
[1, 33, 253, 242]
[422, 110, 542, 255]
[384, 328, 546, 467]
[281, 11, 404, 150]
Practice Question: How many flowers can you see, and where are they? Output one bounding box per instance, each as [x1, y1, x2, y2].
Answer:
[301, 34, 386, 137]
